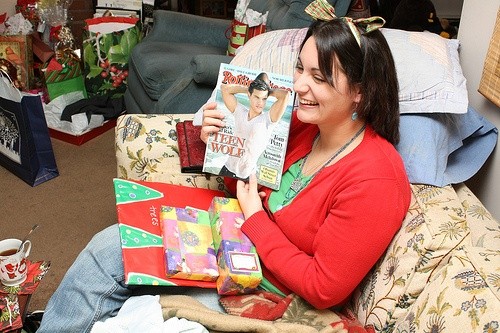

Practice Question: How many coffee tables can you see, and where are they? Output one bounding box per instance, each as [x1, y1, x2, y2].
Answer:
[0, 294, 32, 333]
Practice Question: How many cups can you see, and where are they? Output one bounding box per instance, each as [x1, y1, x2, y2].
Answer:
[0, 238, 32, 286]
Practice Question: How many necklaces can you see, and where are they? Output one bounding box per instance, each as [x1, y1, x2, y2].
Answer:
[275, 122, 369, 211]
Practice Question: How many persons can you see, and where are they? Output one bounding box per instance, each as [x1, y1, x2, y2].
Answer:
[219, 79, 290, 180]
[35, 18, 411, 333]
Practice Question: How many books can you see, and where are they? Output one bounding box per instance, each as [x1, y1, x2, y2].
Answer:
[202, 61, 295, 192]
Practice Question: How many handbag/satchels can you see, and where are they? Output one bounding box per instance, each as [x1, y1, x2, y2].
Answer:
[224, 18, 267, 57]
[43, 50, 87, 101]
[0, 67, 60, 187]
[82, 16, 144, 102]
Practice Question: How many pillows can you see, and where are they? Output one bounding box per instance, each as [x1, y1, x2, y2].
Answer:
[192, 27, 468, 127]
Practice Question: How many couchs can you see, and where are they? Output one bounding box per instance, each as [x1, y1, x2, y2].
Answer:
[124, 0, 351, 114]
[115, 113, 500, 333]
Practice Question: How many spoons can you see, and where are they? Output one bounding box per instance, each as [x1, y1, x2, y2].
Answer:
[16, 224, 40, 253]
